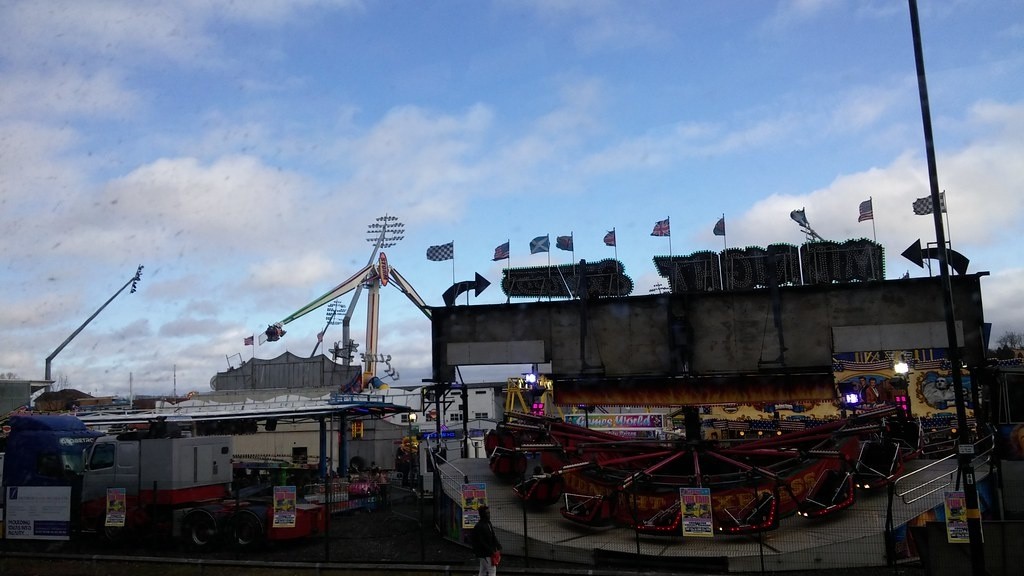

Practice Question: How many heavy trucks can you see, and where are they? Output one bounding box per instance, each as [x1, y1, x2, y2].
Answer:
[0, 414, 106, 545]
[82, 421, 326, 554]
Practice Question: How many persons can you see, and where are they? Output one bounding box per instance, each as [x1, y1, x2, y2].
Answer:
[471, 505, 502, 576]
[516, 466, 542, 494]
[858, 376, 869, 404]
[866, 377, 888, 404]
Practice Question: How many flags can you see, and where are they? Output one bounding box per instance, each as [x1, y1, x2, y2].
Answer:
[791, 210, 806, 226]
[858, 199, 874, 222]
[556, 235, 573, 251]
[427, 241, 453, 261]
[912, 192, 947, 215]
[492, 242, 509, 261]
[530, 236, 549, 254]
[650, 219, 670, 236]
[603, 230, 616, 246]
[713, 218, 725, 236]
[244, 336, 254, 346]
[317, 331, 323, 342]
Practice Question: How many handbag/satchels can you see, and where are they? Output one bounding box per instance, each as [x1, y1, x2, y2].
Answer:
[492, 551, 501, 566]
